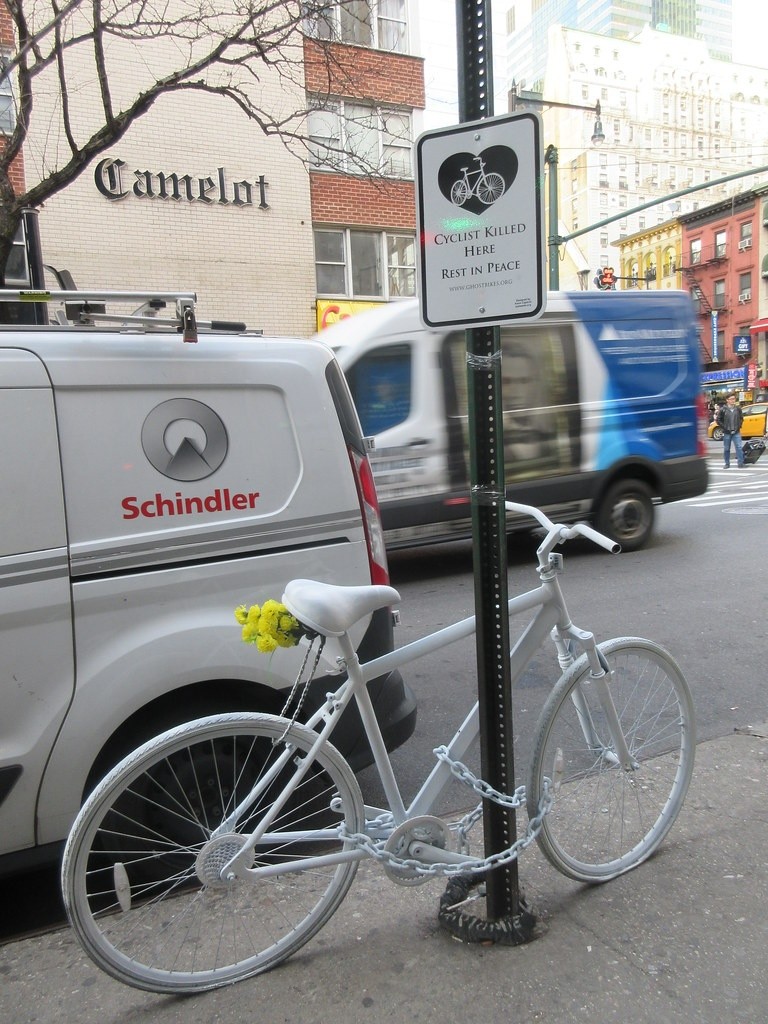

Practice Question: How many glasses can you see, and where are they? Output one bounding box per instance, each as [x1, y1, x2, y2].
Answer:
[728, 398, 736, 400]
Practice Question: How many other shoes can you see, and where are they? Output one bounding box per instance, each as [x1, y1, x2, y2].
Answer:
[738, 464, 749, 468]
[723, 464, 730, 469]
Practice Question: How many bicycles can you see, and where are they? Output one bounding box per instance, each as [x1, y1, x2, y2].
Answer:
[58, 498, 699, 996]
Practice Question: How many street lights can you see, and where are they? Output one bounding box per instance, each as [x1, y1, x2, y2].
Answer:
[508, 77, 605, 147]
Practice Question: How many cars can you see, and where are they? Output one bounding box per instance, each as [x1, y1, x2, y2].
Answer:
[707, 402, 768, 441]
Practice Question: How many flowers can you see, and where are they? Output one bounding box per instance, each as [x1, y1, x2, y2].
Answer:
[234, 600, 315, 654]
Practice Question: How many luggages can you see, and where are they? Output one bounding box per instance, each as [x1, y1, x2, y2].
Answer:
[735, 435, 768, 464]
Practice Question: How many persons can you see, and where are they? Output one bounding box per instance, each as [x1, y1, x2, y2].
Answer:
[716, 394, 749, 469]
[708, 409, 714, 424]
[765, 408, 768, 438]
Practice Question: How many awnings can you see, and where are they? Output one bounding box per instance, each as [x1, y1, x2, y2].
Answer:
[749, 319, 768, 334]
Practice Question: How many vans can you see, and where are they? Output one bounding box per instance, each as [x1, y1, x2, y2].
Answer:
[303, 290, 709, 550]
[0, 290, 418, 866]
